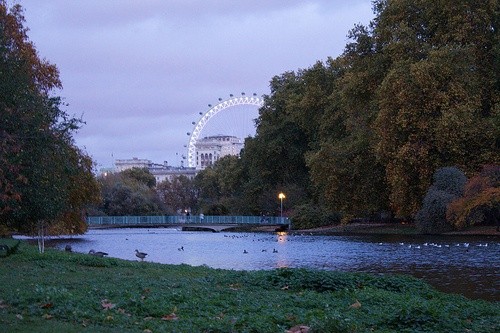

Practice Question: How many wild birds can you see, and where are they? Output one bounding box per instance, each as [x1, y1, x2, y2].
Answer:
[65, 244, 72, 253]
[134, 250, 148, 262]
[88, 248, 108, 257]
[243, 250, 248, 253]
[272, 249, 278, 253]
[178, 246, 184, 252]
[399, 242, 500, 250]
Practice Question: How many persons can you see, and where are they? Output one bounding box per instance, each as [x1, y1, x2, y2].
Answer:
[266, 211, 271, 223]
[199, 212, 205, 222]
[258, 211, 264, 223]
[185, 211, 191, 220]
[271, 210, 278, 223]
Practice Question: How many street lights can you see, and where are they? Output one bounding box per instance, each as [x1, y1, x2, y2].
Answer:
[278, 192, 286, 217]
